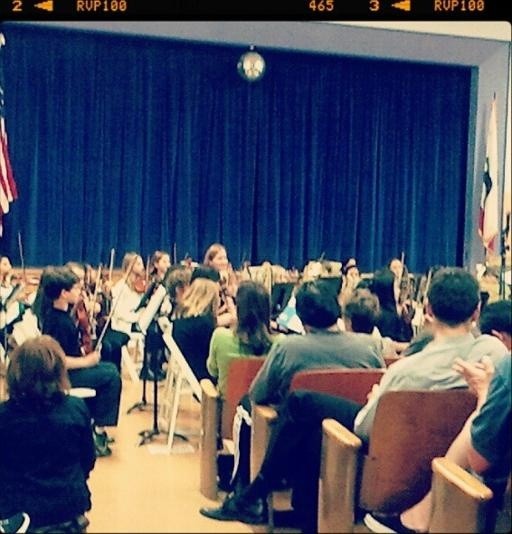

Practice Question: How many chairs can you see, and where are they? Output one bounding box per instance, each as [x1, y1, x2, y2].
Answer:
[201, 356, 266, 498]
[250, 368, 385, 512]
[158, 315, 203, 448]
[316, 391, 477, 534]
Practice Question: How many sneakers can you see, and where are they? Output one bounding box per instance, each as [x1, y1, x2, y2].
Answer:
[0, 512, 31, 534]
[92, 427, 115, 457]
[363, 511, 429, 534]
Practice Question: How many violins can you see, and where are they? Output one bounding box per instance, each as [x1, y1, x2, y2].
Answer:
[75, 286, 93, 356]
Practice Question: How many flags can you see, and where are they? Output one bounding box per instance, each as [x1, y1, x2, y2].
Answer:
[0, 75, 18, 238]
[477, 99, 499, 255]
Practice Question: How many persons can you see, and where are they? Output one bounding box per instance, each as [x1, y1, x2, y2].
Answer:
[1, 257, 122, 456]
[198, 267, 509, 533]
[1, 334, 95, 534]
[233, 279, 386, 495]
[364, 350, 512, 533]
[479, 301, 512, 353]
[206, 281, 274, 492]
[110, 244, 275, 401]
[270, 256, 435, 358]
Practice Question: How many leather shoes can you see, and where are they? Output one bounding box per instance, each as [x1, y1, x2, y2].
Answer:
[199, 495, 270, 526]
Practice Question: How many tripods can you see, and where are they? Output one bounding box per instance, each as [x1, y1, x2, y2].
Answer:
[137, 325, 189, 447]
[126, 347, 160, 414]
